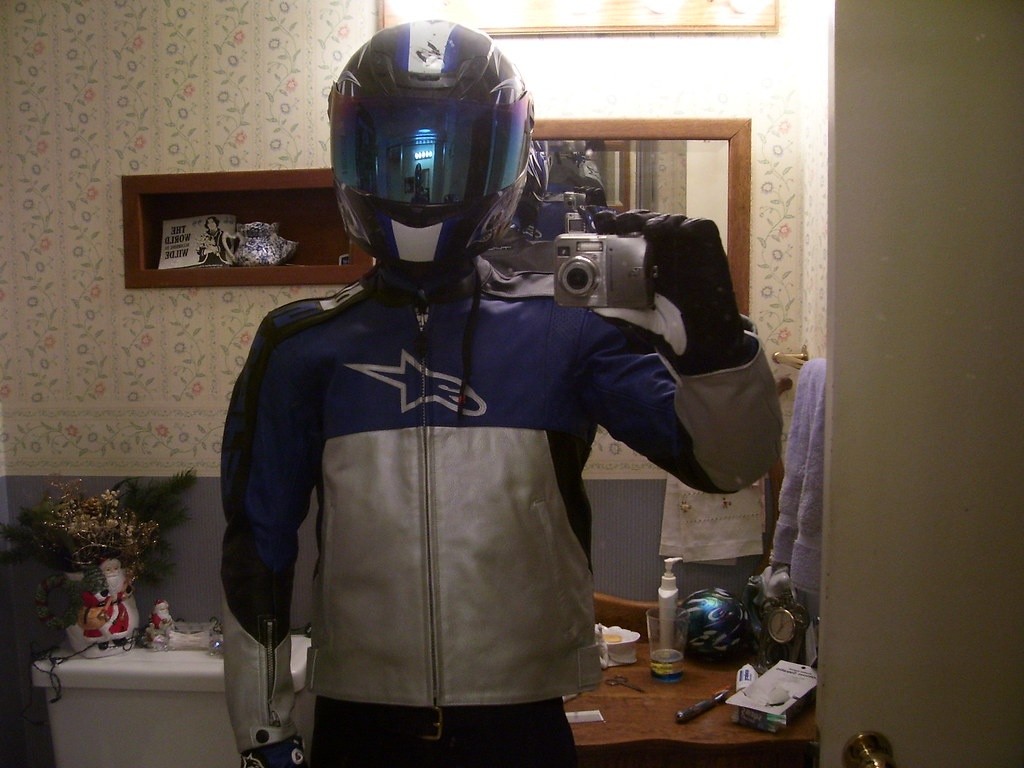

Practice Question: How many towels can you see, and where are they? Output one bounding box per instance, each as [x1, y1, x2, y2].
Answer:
[657, 471, 770, 568]
[768, 355, 829, 601]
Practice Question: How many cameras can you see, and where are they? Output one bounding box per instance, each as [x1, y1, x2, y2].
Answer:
[564, 191, 587, 209]
[553, 233, 658, 310]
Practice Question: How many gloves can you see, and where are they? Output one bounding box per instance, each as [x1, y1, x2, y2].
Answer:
[240, 728, 308, 768]
[574, 183, 607, 231]
[593, 207, 759, 375]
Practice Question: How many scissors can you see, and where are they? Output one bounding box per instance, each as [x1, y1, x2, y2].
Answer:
[604, 675, 645, 693]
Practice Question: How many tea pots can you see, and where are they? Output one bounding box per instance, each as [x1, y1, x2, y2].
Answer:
[221, 222, 299, 267]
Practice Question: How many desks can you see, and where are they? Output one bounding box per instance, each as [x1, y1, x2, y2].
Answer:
[564, 643, 818, 768]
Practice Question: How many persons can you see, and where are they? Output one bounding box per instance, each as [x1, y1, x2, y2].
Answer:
[197, 217, 233, 269]
[218, 20, 786, 767]
[511, 136, 607, 241]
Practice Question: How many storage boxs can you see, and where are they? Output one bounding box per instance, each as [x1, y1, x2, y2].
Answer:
[725, 660, 818, 732]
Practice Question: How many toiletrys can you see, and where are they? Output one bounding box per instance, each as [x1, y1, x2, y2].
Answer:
[657, 555, 683, 651]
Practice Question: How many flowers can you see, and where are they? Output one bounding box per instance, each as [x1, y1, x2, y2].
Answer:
[0, 467, 197, 630]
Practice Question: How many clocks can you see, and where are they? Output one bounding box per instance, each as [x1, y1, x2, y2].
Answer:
[755, 593, 812, 674]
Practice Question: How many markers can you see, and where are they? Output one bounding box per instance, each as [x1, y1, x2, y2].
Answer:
[674, 690, 730, 723]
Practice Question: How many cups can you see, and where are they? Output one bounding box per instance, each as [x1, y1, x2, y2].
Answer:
[646, 608, 689, 682]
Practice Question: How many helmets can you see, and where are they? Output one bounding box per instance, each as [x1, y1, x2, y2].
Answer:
[513, 138, 553, 216]
[326, 18, 534, 293]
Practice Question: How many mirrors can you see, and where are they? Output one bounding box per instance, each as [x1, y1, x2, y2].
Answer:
[509, 117, 751, 318]
